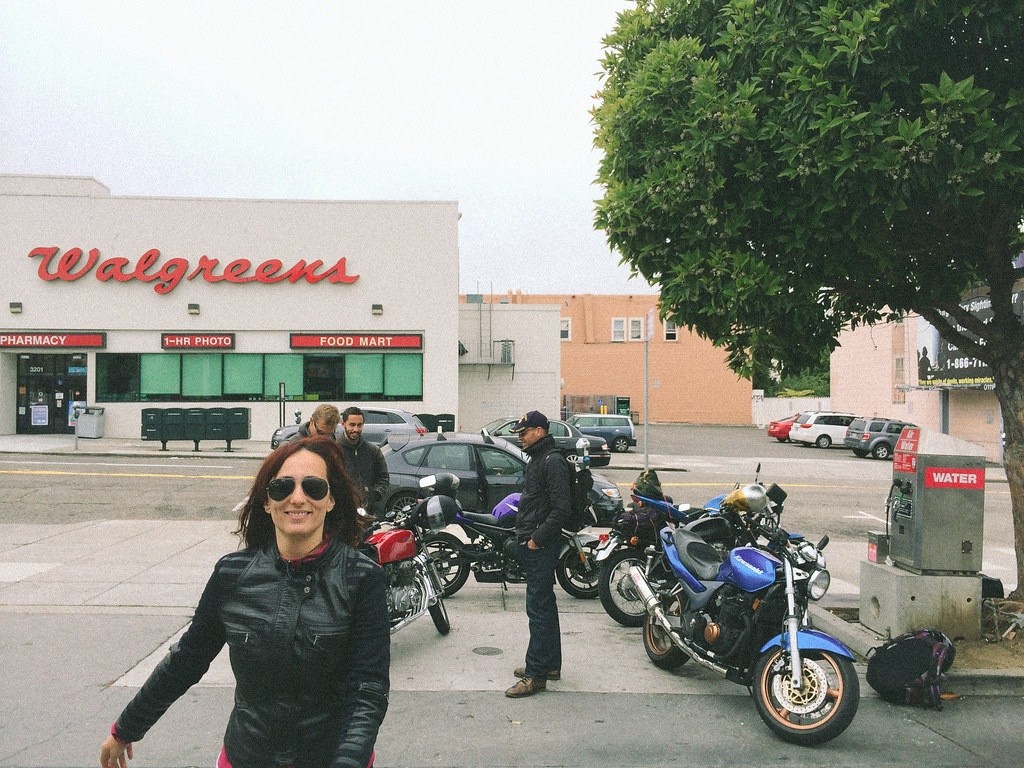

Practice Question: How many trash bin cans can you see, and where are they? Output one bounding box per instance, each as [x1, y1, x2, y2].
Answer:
[631, 410, 639, 425]
[72, 406, 105, 439]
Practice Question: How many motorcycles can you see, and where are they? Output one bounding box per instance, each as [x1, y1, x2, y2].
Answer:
[627, 482, 861, 749]
[418, 459, 614, 601]
[346, 487, 452, 638]
[595, 460, 818, 630]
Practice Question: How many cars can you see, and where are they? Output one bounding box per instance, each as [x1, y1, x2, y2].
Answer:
[270, 407, 428, 461]
[372, 424, 626, 526]
[767, 414, 799, 443]
[474, 415, 612, 472]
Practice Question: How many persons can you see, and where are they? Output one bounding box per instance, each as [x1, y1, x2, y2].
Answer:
[100, 436, 390, 768]
[286, 404, 340, 441]
[335, 406, 390, 515]
[505, 411, 571, 698]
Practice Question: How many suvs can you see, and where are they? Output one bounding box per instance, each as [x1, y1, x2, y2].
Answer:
[562, 413, 639, 452]
[843, 418, 919, 461]
[789, 410, 864, 450]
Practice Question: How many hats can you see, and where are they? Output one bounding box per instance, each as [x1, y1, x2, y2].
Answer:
[508, 410, 549, 433]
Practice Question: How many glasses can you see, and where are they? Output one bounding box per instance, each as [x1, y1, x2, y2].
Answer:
[265, 476, 331, 501]
[519, 425, 538, 437]
[314, 419, 335, 437]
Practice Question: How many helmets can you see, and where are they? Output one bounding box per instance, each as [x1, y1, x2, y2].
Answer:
[412, 495, 458, 532]
[428, 472, 460, 497]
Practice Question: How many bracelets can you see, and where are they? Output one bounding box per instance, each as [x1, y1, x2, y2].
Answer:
[111, 724, 132, 745]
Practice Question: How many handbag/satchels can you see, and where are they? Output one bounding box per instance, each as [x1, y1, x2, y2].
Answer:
[632, 469, 663, 498]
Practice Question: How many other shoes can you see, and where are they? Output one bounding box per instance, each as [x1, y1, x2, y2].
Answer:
[514, 666, 560, 681]
[505, 677, 546, 698]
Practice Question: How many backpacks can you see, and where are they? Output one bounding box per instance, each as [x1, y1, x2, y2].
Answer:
[539, 449, 588, 533]
[864, 629, 955, 713]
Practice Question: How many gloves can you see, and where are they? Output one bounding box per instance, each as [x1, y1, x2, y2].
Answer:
[368, 490, 381, 504]
